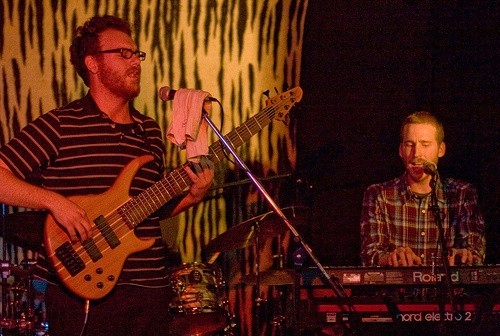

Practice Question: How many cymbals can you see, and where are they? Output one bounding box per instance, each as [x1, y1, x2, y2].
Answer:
[205, 204, 310, 253]
[1, 208, 49, 254]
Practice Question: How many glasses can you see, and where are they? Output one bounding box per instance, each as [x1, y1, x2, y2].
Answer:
[96, 48, 146, 61]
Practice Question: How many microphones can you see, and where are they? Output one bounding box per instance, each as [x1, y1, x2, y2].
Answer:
[423, 161, 439, 175]
[158, 85, 214, 103]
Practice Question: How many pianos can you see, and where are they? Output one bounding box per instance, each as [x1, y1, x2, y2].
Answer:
[238, 261, 500, 336]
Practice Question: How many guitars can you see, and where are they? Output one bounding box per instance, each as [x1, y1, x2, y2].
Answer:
[45, 84, 304, 300]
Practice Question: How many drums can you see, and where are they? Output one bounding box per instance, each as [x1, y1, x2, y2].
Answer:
[167, 261, 236, 336]
[0, 285, 33, 328]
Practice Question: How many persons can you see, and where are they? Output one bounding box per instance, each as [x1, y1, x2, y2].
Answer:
[0, 15, 215, 336]
[360, 111, 486, 295]
